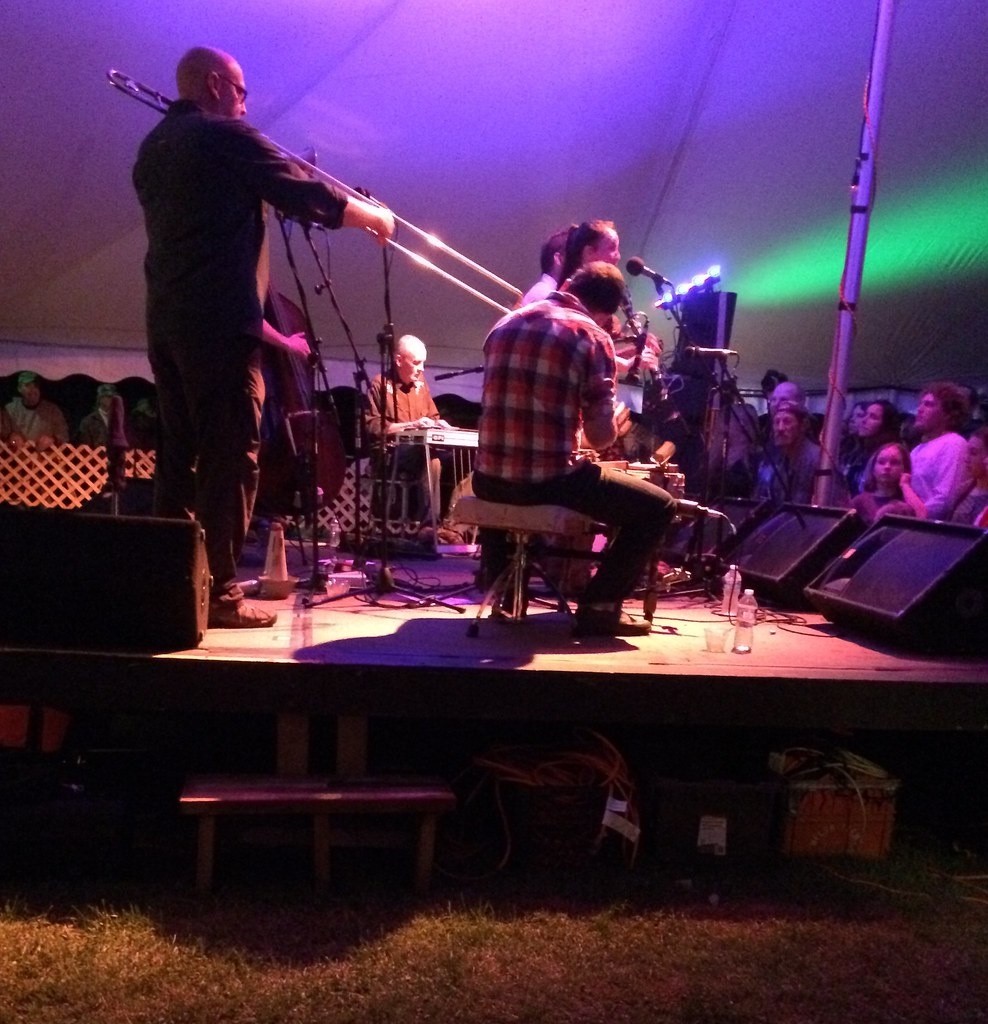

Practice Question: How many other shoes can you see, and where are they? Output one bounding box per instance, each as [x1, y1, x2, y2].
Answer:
[576, 603, 653, 636]
[208, 583, 278, 627]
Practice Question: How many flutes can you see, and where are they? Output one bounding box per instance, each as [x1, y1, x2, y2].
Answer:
[619, 286, 680, 424]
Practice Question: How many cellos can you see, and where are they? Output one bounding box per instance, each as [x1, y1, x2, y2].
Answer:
[253, 278, 347, 566]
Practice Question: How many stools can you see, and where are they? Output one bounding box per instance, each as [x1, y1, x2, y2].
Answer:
[455, 494, 591, 629]
[377, 478, 422, 525]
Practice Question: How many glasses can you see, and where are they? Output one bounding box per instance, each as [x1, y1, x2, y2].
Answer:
[217, 74, 248, 103]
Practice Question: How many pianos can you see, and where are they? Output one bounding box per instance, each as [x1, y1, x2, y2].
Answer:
[380, 427, 479, 561]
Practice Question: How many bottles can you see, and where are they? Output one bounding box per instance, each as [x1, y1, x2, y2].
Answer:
[722, 565, 741, 617]
[733, 589, 758, 651]
[330, 516, 341, 547]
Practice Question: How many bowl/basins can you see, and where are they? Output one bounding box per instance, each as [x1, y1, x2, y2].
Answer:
[262, 576, 297, 598]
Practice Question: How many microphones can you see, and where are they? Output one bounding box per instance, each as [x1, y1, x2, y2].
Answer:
[685, 346, 739, 358]
[626, 323, 648, 385]
[310, 222, 325, 231]
[354, 187, 389, 210]
[626, 256, 674, 286]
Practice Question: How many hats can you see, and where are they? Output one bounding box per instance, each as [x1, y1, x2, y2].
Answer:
[129, 398, 158, 419]
[18, 371, 39, 384]
[95, 383, 120, 397]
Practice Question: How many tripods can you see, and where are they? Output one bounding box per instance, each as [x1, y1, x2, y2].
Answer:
[272, 209, 468, 612]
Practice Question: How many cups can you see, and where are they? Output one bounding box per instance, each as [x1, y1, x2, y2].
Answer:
[704, 627, 730, 653]
[327, 579, 350, 598]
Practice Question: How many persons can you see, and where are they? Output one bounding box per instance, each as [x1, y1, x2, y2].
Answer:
[0, 370, 133, 449]
[518, 219, 988, 529]
[468, 260, 674, 633]
[136, 46, 442, 629]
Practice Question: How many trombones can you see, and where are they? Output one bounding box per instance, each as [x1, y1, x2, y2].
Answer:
[106, 66, 525, 314]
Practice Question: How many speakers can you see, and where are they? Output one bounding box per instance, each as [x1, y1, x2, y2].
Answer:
[661, 497, 988, 657]
[0, 506, 213, 654]
